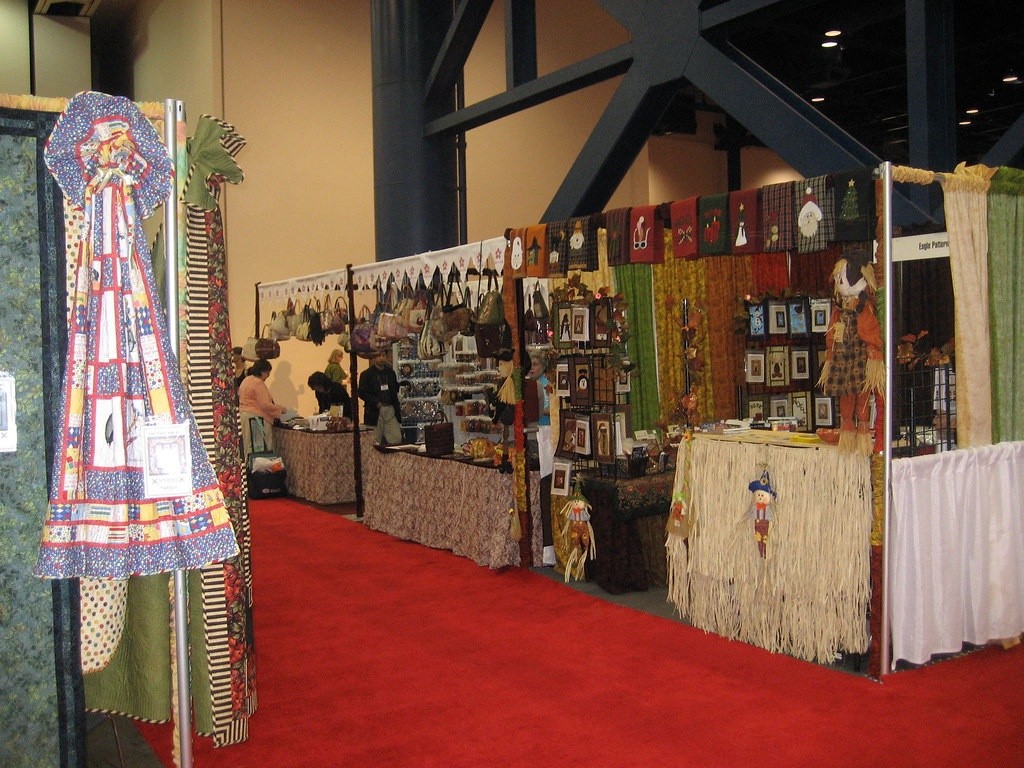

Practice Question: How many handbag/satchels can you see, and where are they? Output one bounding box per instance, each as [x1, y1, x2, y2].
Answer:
[424, 410, 455, 457]
[249, 453, 275, 470]
[240, 271, 548, 361]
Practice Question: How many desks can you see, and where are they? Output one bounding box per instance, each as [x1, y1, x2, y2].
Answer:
[572, 466, 678, 596]
[271, 418, 377, 505]
[363, 438, 544, 570]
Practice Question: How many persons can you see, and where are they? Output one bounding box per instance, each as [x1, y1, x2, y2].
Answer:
[325, 349, 351, 392]
[359, 351, 402, 445]
[492, 316, 516, 473]
[232, 347, 247, 386]
[933, 408, 958, 440]
[560, 472, 596, 582]
[816, 253, 886, 455]
[496, 349, 553, 478]
[240, 360, 286, 497]
[670, 493, 687, 527]
[747, 462, 777, 559]
[308, 371, 352, 423]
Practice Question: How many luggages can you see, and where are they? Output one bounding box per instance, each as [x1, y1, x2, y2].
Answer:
[246, 416, 287, 499]
